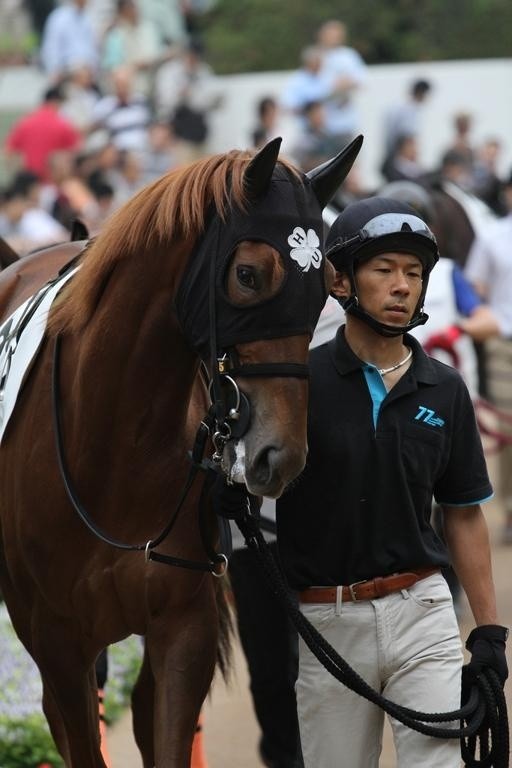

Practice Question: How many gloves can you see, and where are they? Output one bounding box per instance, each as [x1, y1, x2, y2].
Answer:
[466, 625, 509, 689]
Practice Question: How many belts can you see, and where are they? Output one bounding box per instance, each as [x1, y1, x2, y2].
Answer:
[298, 567, 434, 602]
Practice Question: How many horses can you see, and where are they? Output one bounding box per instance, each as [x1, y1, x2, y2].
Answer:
[0, 130, 367, 768]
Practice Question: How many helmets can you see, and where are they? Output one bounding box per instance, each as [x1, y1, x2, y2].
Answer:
[324, 198, 439, 272]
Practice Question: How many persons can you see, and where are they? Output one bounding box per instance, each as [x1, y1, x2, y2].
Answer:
[0, 2, 221, 268]
[278, 199, 508, 768]
[251, 18, 512, 545]
[231, 440, 306, 766]
